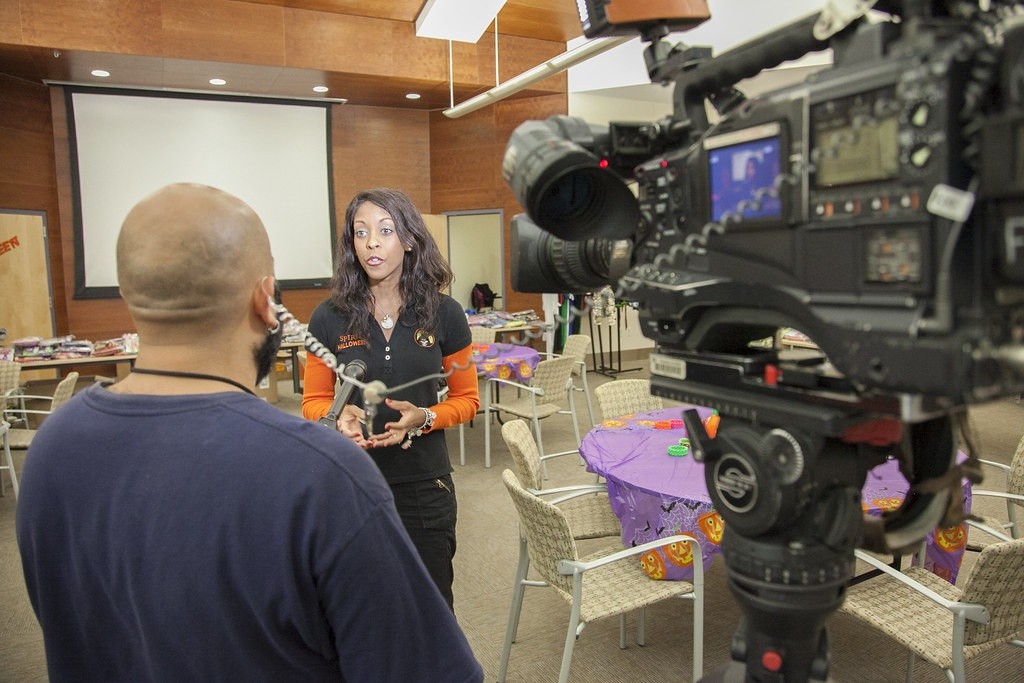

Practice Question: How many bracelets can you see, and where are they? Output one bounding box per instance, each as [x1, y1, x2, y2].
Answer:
[407, 407, 437, 440]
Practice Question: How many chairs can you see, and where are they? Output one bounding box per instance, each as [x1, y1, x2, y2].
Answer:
[442, 332, 706, 683]
[0, 361, 80, 498]
[837, 435, 1024, 683]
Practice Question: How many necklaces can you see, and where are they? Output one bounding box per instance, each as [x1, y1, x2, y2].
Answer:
[370, 293, 400, 329]
[129, 367, 256, 395]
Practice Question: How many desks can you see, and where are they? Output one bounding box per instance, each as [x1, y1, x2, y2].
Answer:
[21, 355, 138, 383]
[278, 342, 306, 393]
[579, 406, 971, 513]
[458, 342, 538, 464]
[468, 325, 541, 428]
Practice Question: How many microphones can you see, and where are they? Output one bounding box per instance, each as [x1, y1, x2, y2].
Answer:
[317, 359, 368, 432]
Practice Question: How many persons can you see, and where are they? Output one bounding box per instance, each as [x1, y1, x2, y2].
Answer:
[301, 188, 480, 616]
[14, 181, 485, 683]
[730, 158, 765, 221]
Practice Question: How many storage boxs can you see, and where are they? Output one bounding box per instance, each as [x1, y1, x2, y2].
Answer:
[256, 367, 280, 404]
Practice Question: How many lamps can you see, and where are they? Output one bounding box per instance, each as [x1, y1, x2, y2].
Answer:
[440, 10, 639, 119]
